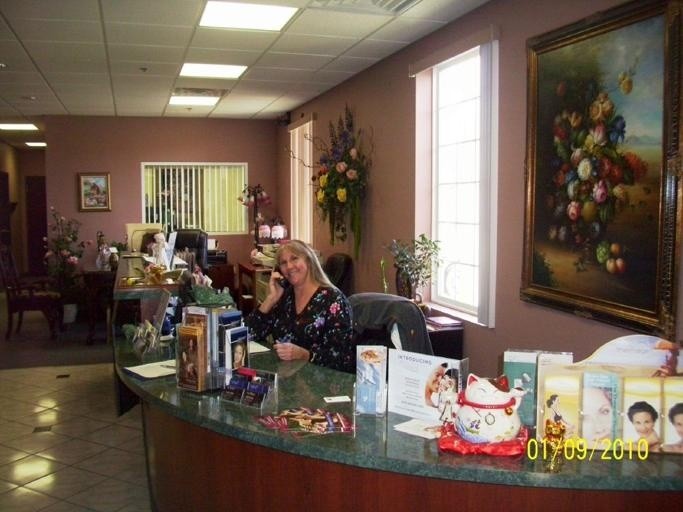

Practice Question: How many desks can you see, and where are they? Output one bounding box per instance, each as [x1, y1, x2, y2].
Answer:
[205, 265, 234, 296]
[56, 272, 140, 345]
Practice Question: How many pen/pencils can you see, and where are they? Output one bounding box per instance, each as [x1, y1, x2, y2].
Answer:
[160, 364, 177, 369]
[280, 334, 291, 343]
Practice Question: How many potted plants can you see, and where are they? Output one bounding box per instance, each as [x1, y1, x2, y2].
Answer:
[385, 233, 440, 303]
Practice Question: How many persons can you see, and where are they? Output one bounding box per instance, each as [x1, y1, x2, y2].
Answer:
[585, 385, 612, 446]
[186, 339, 196, 364]
[233, 342, 244, 366]
[438, 368, 457, 394]
[662, 404, 683, 453]
[425, 363, 446, 409]
[244, 238, 353, 372]
[626, 401, 661, 451]
[181, 352, 194, 379]
[547, 395, 576, 440]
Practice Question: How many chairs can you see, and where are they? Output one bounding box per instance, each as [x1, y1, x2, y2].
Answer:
[323, 253, 351, 293]
[348, 292, 436, 357]
[0, 249, 61, 341]
[168, 229, 209, 278]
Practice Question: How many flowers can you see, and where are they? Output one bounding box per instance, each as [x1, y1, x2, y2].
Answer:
[43, 206, 94, 283]
[546, 64, 648, 276]
[286, 109, 370, 260]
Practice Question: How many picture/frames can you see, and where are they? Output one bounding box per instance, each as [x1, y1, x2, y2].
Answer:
[76, 171, 111, 213]
[520, 0, 683, 345]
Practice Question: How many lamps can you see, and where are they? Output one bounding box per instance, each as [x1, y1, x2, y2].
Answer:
[237, 185, 288, 250]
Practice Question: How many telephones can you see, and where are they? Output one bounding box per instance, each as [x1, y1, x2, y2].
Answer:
[273, 264, 290, 289]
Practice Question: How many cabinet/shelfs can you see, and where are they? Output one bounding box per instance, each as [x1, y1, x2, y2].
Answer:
[238, 258, 273, 315]
[424, 320, 464, 358]
